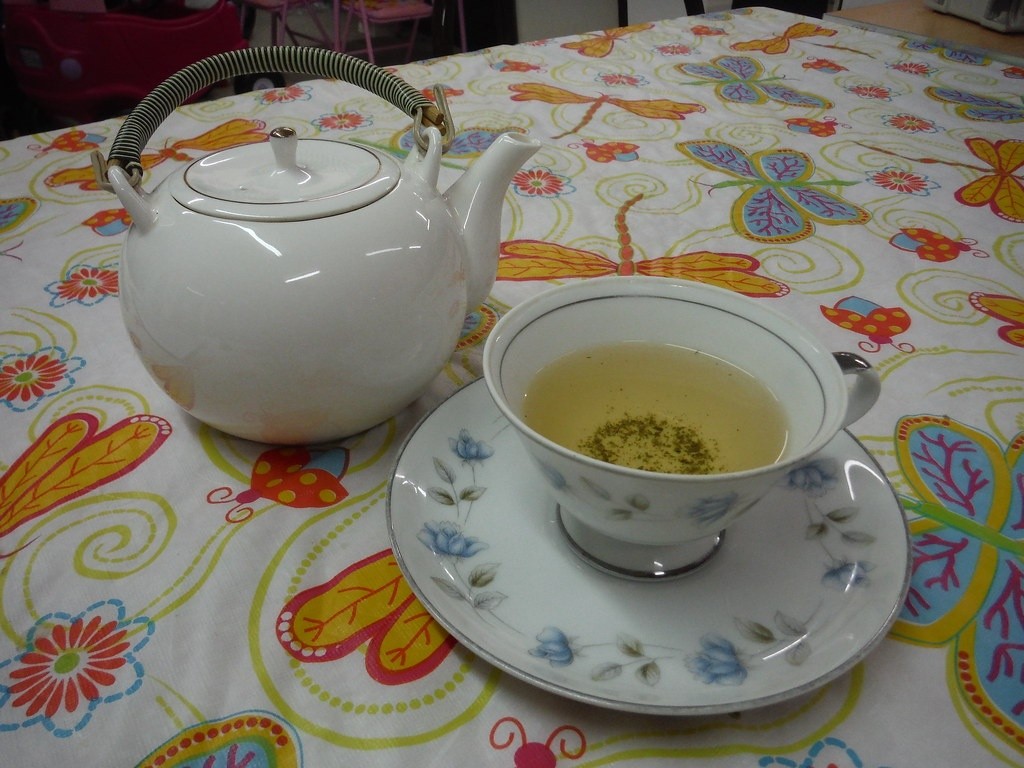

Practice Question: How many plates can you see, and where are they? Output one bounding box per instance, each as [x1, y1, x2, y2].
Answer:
[386, 377, 914, 716]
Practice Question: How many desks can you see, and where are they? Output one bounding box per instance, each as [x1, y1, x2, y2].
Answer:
[0, 8, 1024, 768]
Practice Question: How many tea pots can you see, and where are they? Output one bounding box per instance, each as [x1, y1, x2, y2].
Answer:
[91, 47, 542, 445]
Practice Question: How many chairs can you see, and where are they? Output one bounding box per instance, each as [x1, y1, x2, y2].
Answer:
[240, 0, 434, 65]
[0, 0, 249, 128]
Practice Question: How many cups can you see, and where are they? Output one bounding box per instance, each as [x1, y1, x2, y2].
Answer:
[481, 276, 881, 581]
